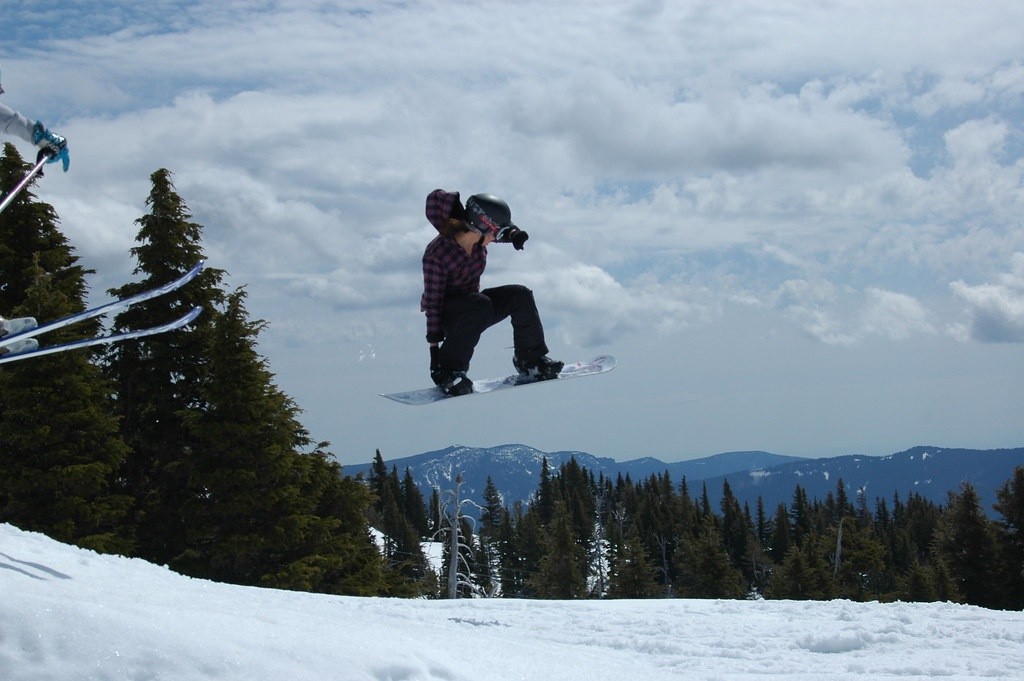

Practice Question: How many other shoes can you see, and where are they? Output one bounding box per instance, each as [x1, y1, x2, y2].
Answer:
[440, 369, 472, 394]
[512, 353, 564, 378]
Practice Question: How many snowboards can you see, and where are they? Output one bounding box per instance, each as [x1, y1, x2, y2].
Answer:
[379, 355, 618, 407]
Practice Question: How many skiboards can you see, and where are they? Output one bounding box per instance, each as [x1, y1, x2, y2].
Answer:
[0, 307, 201, 363]
[0, 258, 206, 347]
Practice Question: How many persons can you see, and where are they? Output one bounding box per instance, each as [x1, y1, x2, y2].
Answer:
[0, 84, 67, 159]
[421, 189, 564, 395]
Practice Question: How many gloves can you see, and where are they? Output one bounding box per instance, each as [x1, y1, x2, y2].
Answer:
[31, 120, 69, 171]
[430, 346, 443, 385]
[508, 226, 529, 250]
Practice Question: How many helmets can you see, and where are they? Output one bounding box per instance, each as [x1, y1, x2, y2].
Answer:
[466, 193, 514, 232]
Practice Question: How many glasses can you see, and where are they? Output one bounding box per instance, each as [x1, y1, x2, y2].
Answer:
[493, 227, 510, 240]
